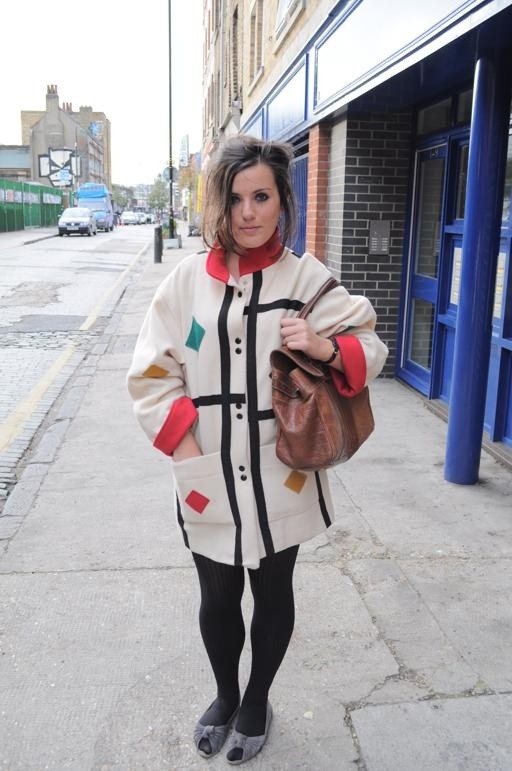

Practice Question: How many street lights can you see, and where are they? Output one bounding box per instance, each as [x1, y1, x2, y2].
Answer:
[269, 277, 375, 472]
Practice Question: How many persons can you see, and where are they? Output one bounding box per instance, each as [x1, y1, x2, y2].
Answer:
[121, 132, 391, 770]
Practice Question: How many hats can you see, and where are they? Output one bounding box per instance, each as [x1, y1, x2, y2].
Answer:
[121, 206, 156, 225]
[57, 206, 96, 238]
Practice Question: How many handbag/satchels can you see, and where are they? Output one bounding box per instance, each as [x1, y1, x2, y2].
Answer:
[321, 338, 341, 366]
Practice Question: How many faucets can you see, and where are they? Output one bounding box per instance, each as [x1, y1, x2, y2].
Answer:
[193, 696, 241, 759]
[226, 700, 273, 766]
